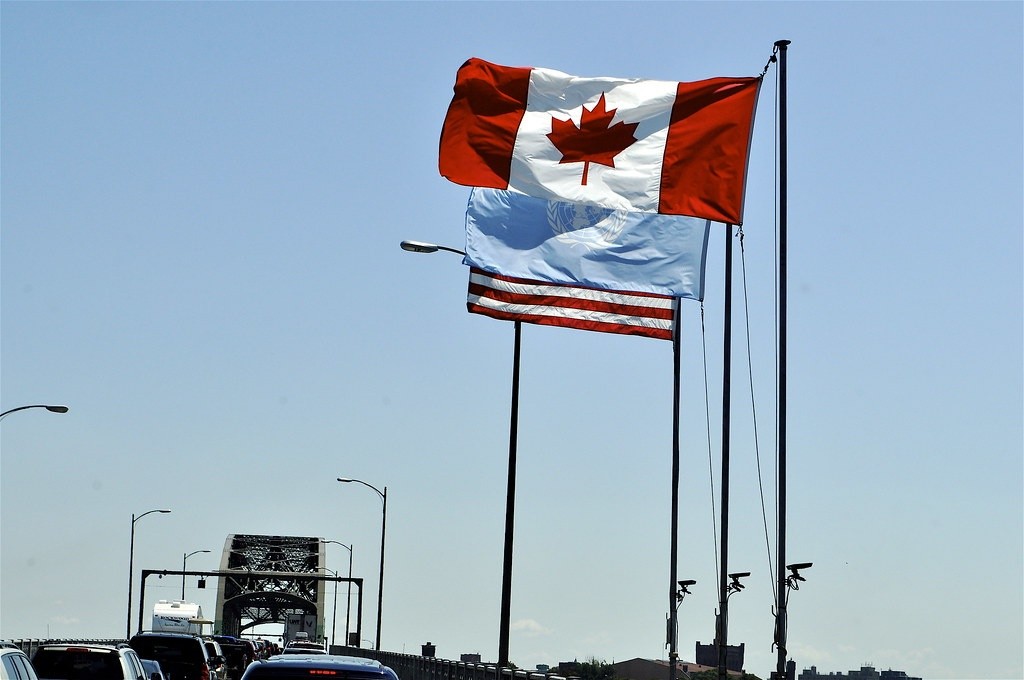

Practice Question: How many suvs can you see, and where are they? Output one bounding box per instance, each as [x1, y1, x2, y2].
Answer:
[128, 630, 225, 680]
[31, 643, 148, 680]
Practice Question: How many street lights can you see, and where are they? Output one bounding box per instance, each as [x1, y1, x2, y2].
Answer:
[182, 550, 211, 600]
[315, 567, 338, 645]
[400, 240, 525, 680]
[337, 477, 387, 651]
[321, 540, 353, 647]
[126, 509, 171, 639]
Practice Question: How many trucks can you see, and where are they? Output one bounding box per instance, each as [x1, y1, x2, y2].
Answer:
[282, 614, 317, 649]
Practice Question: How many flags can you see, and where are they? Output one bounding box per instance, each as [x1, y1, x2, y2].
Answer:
[467, 264, 678, 339]
[438, 57, 763, 226]
[461, 187, 712, 302]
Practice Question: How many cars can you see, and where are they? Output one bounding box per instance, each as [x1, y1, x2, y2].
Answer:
[0, 640, 21, 650]
[240, 655, 400, 680]
[140, 659, 167, 680]
[210, 631, 328, 680]
[0, 648, 41, 680]
